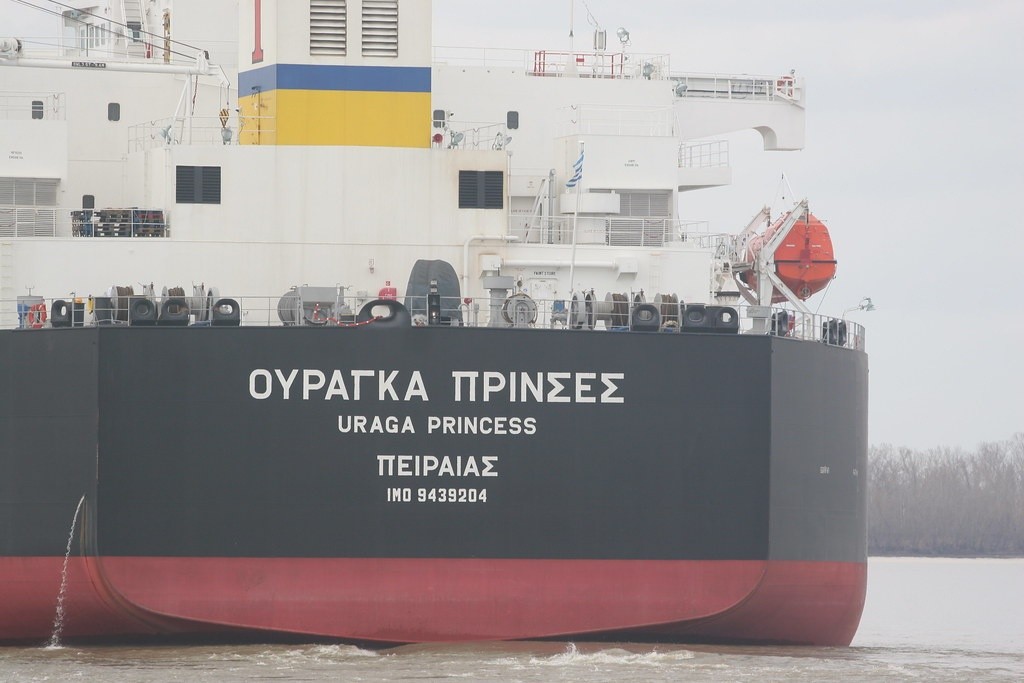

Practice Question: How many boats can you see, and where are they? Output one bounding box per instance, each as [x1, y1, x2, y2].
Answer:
[0, 1, 872, 647]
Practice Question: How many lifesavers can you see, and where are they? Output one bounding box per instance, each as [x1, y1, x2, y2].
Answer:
[29, 304, 48, 329]
